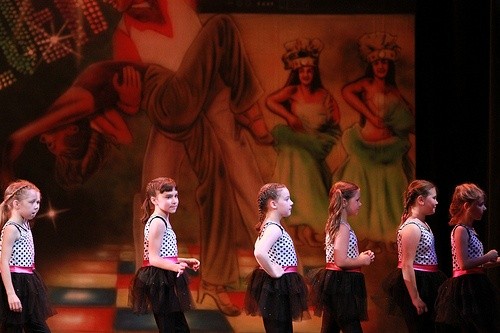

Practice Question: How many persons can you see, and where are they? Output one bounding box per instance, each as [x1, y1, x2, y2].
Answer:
[437, 183, 500, 333]
[0, 178, 57, 333]
[243, 182, 311, 333]
[381, 179, 442, 333]
[308, 180, 375, 333]
[127, 177, 201, 333]
